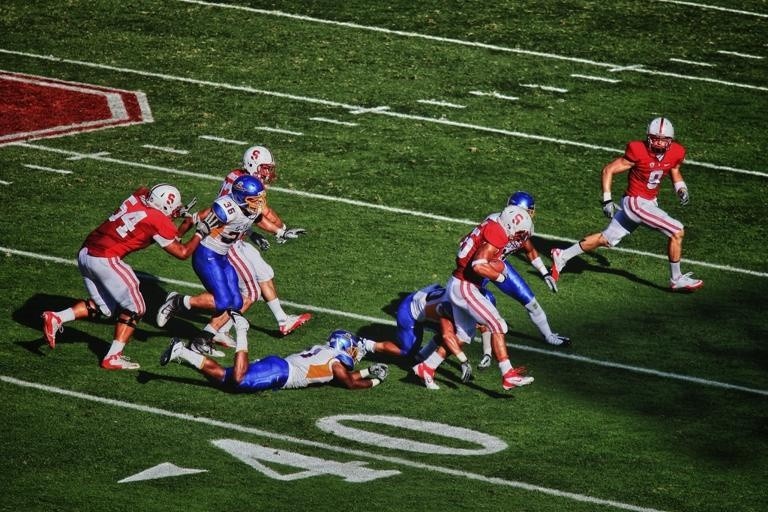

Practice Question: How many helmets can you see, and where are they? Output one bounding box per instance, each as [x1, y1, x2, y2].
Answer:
[232, 174, 267, 218]
[242, 144, 277, 189]
[147, 181, 181, 216]
[500, 192, 535, 249]
[647, 117, 674, 154]
[327, 328, 365, 371]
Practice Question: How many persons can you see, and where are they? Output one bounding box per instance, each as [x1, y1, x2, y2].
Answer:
[549, 114, 706, 292]
[356, 282, 498, 384]
[209, 144, 314, 349]
[160, 329, 389, 395]
[476, 191, 573, 369]
[155, 174, 308, 359]
[410, 204, 536, 390]
[41, 181, 219, 371]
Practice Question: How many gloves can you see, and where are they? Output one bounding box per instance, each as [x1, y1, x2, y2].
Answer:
[249, 230, 269, 251]
[460, 359, 472, 383]
[178, 197, 197, 218]
[368, 363, 390, 380]
[601, 201, 624, 220]
[478, 355, 491, 369]
[277, 224, 307, 244]
[677, 187, 691, 205]
[197, 215, 219, 236]
[544, 273, 558, 294]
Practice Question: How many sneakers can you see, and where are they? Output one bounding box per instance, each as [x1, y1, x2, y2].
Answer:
[157, 290, 181, 326]
[102, 352, 140, 371]
[227, 306, 251, 331]
[278, 313, 310, 336]
[191, 334, 236, 359]
[42, 311, 63, 349]
[545, 334, 570, 346]
[413, 362, 439, 390]
[355, 336, 367, 361]
[160, 334, 183, 366]
[670, 275, 702, 291]
[503, 374, 535, 391]
[551, 248, 564, 279]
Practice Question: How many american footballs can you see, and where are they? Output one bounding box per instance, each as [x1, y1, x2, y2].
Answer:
[487, 259, 506, 275]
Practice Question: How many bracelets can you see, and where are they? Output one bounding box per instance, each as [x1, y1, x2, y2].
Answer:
[456, 352, 468, 363]
[496, 273, 505, 284]
[282, 222, 287, 231]
[600, 191, 612, 202]
[481, 331, 493, 356]
[358, 367, 370, 378]
[371, 377, 382, 388]
[530, 256, 549, 276]
[275, 229, 285, 239]
[674, 181, 688, 192]
